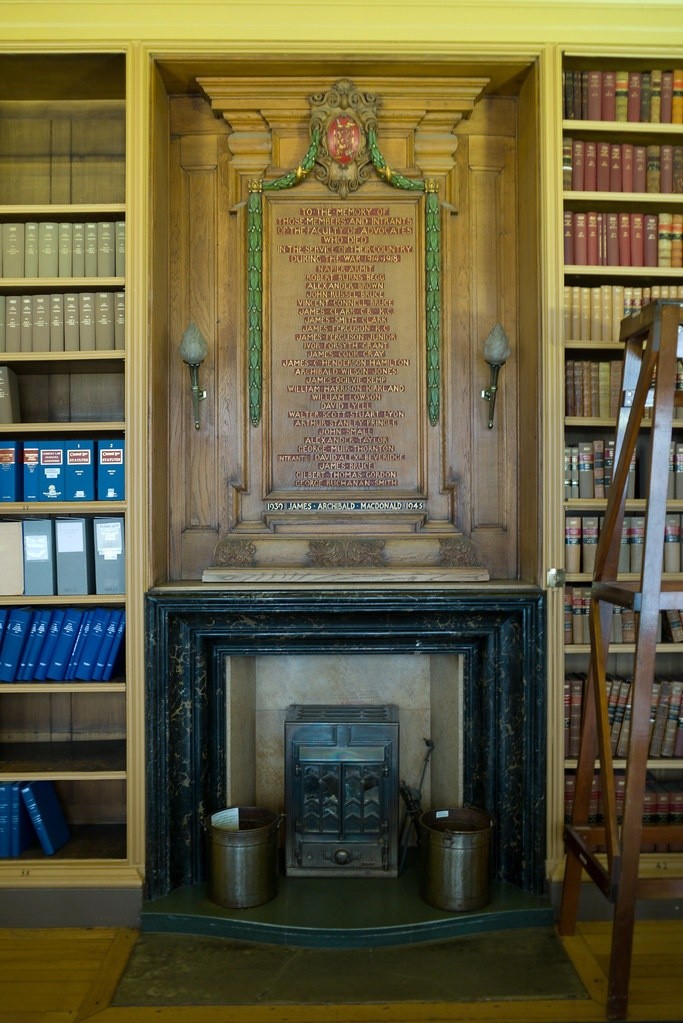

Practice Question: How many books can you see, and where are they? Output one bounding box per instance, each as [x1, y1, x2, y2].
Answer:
[1, 219, 126, 860]
[561, 65, 683, 855]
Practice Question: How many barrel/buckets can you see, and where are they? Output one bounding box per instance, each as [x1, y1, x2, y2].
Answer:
[421, 806, 500, 913]
[203, 805, 288, 909]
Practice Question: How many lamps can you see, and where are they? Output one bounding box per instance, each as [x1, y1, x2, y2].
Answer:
[179, 321, 209, 431]
[481, 324, 511, 430]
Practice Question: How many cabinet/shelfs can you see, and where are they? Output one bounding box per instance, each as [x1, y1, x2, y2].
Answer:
[0, 34, 683, 888]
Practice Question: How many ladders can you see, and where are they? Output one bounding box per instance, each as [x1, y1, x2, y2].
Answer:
[556, 300, 683, 1023]
